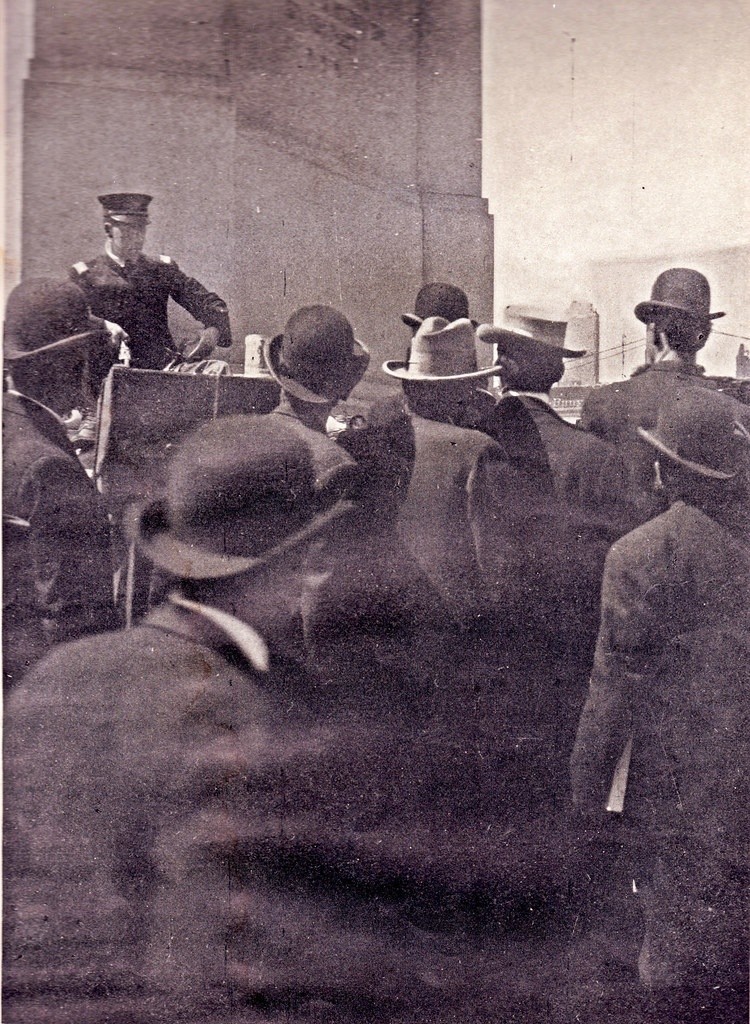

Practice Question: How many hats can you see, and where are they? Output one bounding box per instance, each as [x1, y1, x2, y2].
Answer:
[382, 316, 503, 382]
[476, 304, 587, 357]
[401, 282, 482, 329]
[638, 388, 750, 480]
[132, 416, 359, 579]
[96, 193, 154, 225]
[3, 277, 112, 360]
[263, 305, 371, 403]
[634, 268, 726, 324]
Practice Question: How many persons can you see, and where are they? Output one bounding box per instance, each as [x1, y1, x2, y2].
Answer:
[6, 265, 749, 1024]
[55, 190, 230, 404]
